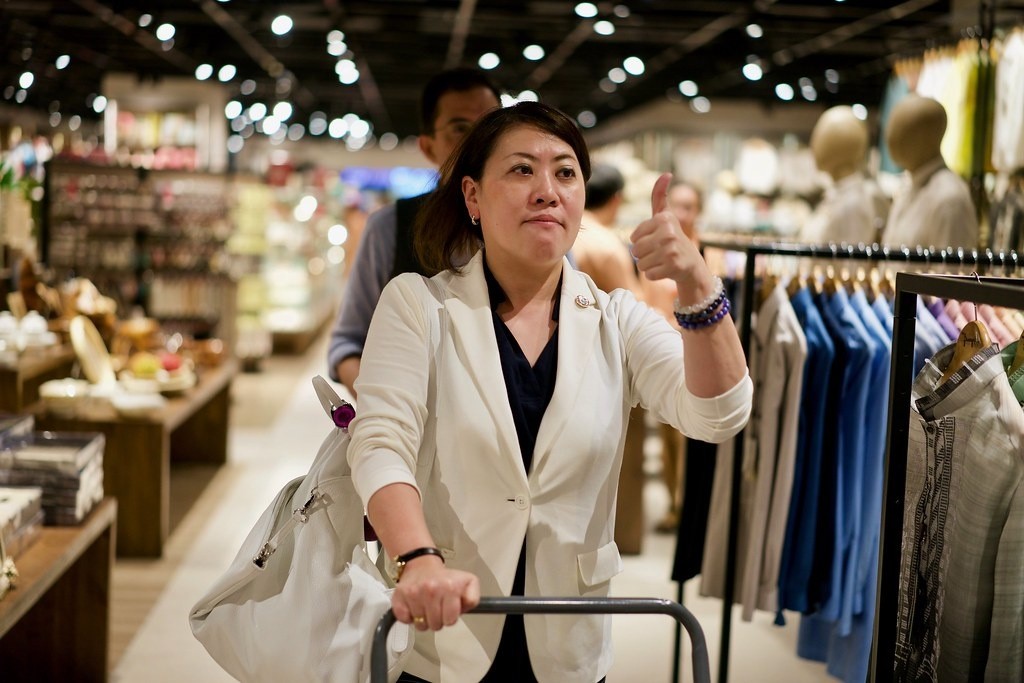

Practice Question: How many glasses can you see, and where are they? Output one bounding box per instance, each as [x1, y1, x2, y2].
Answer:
[434, 121, 474, 137]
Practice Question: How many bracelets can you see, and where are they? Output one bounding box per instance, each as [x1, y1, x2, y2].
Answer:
[674, 288, 726, 318]
[677, 297, 732, 330]
[675, 276, 723, 312]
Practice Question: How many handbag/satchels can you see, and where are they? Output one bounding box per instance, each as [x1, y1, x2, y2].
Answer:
[189, 278, 447, 683]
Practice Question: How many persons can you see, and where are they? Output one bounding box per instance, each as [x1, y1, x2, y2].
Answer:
[566, 164, 643, 552]
[651, 178, 725, 531]
[343, 102, 756, 683]
[327, 65, 505, 406]
[883, 95, 978, 255]
[807, 105, 887, 249]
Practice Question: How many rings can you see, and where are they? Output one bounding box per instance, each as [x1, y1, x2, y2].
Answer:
[413, 617, 425, 623]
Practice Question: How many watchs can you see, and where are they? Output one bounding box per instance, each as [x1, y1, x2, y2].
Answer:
[391, 547, 446, 583]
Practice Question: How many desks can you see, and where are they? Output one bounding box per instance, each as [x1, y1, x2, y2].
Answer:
[1, 495, 118, 683]
[34, 357, 239, 557]
[0, 343, 76, 410]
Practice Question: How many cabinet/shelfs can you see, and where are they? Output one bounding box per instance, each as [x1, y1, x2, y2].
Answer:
[44, 161, 344, 369]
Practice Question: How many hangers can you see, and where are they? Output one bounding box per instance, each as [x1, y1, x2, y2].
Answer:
[698, 230, 1024, 390]
[887, 20, 1023, 93]
